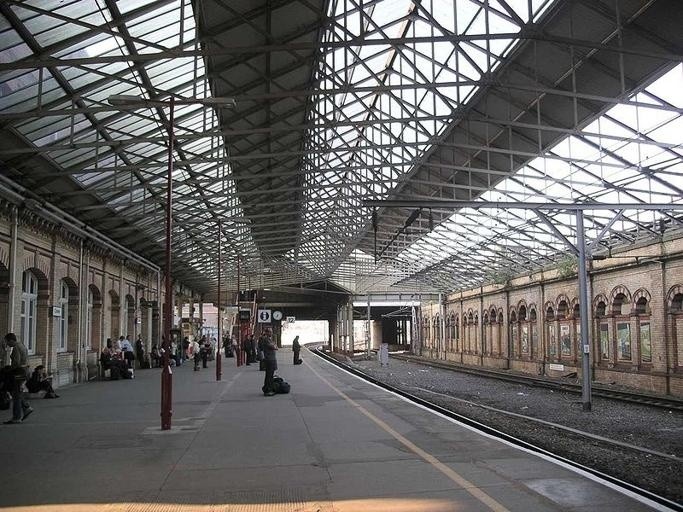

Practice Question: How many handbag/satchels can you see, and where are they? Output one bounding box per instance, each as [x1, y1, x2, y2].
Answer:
[269, 375, 291, 394]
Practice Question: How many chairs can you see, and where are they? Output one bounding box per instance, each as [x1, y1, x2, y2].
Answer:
[100, 358, 120, 380]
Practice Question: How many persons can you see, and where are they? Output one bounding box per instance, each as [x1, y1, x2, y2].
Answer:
[2, 332, 33, 425]
[100, 328, 267, 381]
[264, 328, 278, 384]
[292, 335, 301, 365]
[26, 365, 60, 399]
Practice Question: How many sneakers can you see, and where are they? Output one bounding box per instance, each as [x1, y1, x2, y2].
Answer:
[264, 390, 275, 396]
[20, 405, 35, 422]
[2, 415, 22, 424]
